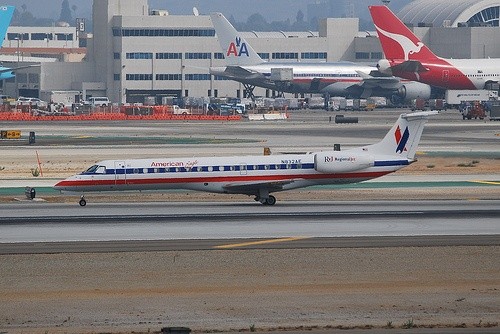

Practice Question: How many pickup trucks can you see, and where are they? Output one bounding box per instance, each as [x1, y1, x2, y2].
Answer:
[171, 105, 190, 115]
[9, 96, 45, 107]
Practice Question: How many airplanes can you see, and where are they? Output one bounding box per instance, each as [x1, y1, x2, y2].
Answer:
[208, 5, 500, 106]
[52, 110, 439, 206]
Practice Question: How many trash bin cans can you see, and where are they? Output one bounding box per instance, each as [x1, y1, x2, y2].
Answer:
[263, 147, 270, 155]
[334, 143, 340, 151]
[29, 131, 36, 144]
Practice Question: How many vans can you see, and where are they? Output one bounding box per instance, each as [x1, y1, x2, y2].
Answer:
[88, 96, 109, 108]
